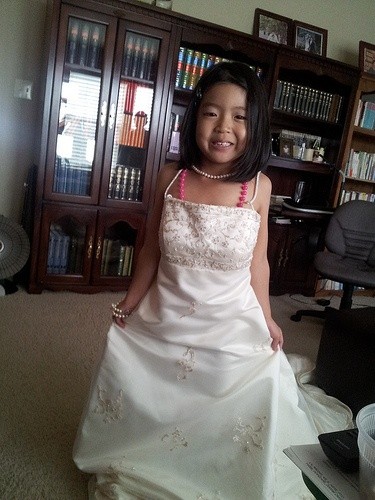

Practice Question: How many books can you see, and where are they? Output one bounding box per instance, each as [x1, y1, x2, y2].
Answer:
[268, 195, 291, 224]
[345, 148, 375, 181]
[339, 190, 375, 205]
[166, 113, 183, 153]
[114, 80, 149, 148]
[175, 46, 262, 92]
[354, 100, 375, 130]
[52, 157, 92, 196]
[66, 19, 106, 69]
[273, 80, 343, 123]
[108, 164, 144, 201]
[122, 36, 159, 82]
[271, 129, 321, 158]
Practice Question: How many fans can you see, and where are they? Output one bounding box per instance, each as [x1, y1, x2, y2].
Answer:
[0, 215, 29, 282]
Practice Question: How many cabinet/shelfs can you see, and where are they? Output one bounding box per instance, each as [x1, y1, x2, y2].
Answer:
[29, 0, 174, 296]
[310, 71, 375, 297]
[155, 10, 361, 293]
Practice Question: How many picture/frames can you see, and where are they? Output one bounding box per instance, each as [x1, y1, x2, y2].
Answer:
[253, 7, 292, 47]
[359, 41, 375, 72]
[291, 20, 328, 56]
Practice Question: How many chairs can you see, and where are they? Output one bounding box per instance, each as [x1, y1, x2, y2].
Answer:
[290, 197, 375, 327]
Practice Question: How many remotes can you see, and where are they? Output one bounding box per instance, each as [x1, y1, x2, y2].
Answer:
[317, 428, 375, 474]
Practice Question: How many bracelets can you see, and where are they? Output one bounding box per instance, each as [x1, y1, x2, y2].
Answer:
[111, 302, 130, 318]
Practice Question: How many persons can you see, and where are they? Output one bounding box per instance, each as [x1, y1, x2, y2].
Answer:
[74, 59, 355, 500]
[283, 144, 290, 155]
[304, 34, 319, 54]
[267, 30, 280, 43]
[46, 226, 134, 277]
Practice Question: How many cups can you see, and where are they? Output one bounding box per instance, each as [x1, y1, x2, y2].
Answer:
[356, 403, 375, 500]
[292, 145, 325, 163]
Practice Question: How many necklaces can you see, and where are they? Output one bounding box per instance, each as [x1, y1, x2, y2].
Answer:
[178, 169, 248, 208]
[191, 164, 240, 179]
